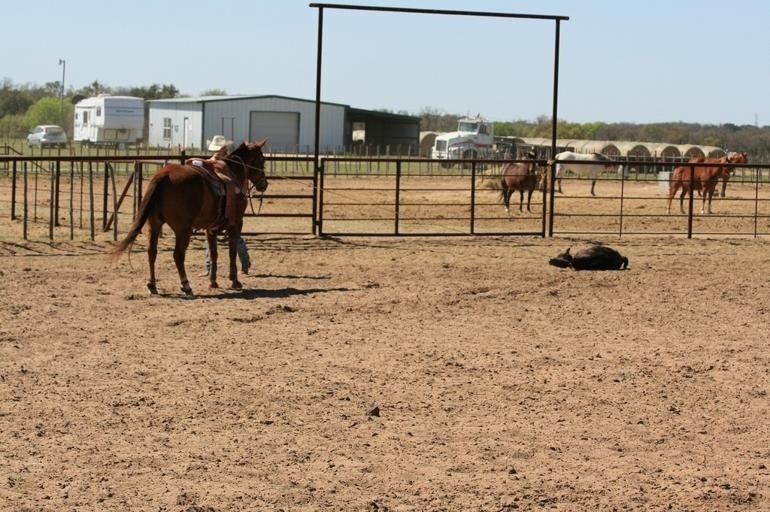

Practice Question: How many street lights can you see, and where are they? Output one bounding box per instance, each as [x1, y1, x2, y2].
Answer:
[58, 58, 65, 96]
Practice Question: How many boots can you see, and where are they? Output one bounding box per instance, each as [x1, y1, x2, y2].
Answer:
[198, 271, 209, 277]
[238, 269, 248, 275]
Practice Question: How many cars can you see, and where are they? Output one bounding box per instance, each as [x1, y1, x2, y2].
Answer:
[26, 125, 68, 150]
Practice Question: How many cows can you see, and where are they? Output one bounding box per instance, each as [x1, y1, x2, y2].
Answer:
[548, 246, 629, 271]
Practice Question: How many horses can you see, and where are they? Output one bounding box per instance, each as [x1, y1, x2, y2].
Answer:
[687, 151, 749, 198]
[496, 158, 539, 214]
[104, 135, 269, 296]
[666, 156, 734, 216]
[554, 151, 617, 196]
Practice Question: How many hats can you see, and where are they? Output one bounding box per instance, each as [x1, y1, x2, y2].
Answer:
[206, 135, 233, 151]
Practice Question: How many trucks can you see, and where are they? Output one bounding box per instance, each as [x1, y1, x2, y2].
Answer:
[431, 113, 574, 171]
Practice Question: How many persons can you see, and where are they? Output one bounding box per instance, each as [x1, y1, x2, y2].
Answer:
[198, 135, 251, 277]
